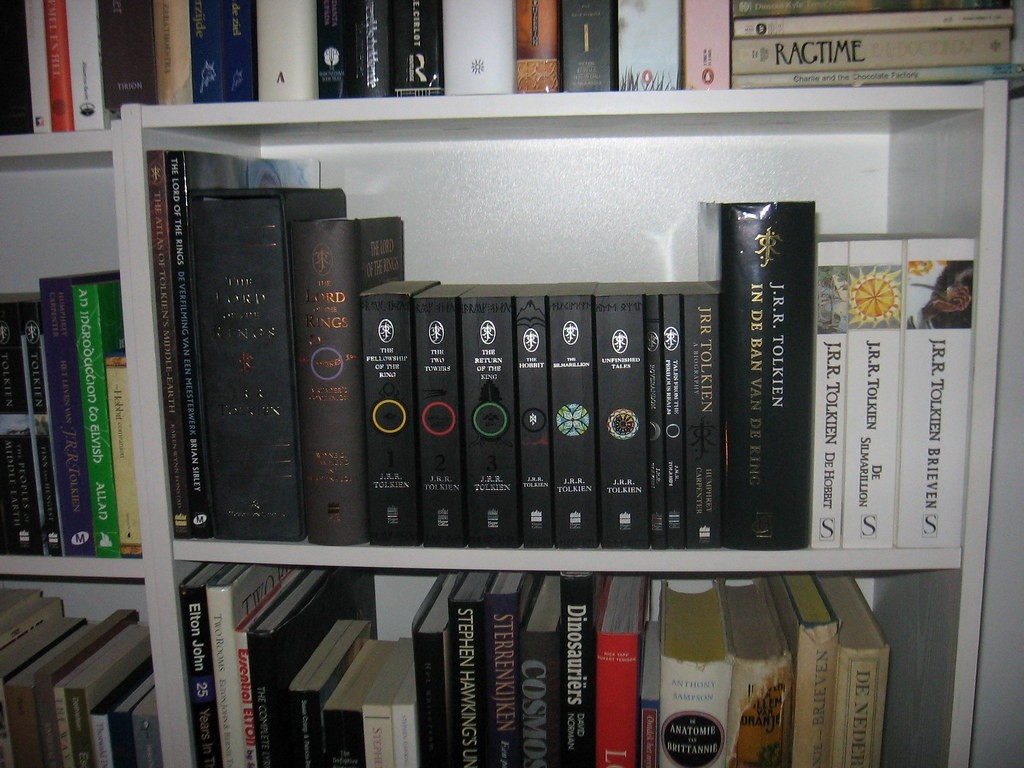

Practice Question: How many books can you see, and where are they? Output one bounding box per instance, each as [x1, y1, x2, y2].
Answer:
[0, 0, 1024, 137]
[0, 587, 165, 768]
[0, 270, 142, 559]
[143, 147, 972, 554]
[177, 562, 890, 767]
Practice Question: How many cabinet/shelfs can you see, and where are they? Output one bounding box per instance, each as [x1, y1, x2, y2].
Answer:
[0, 80, 1011, 768]
